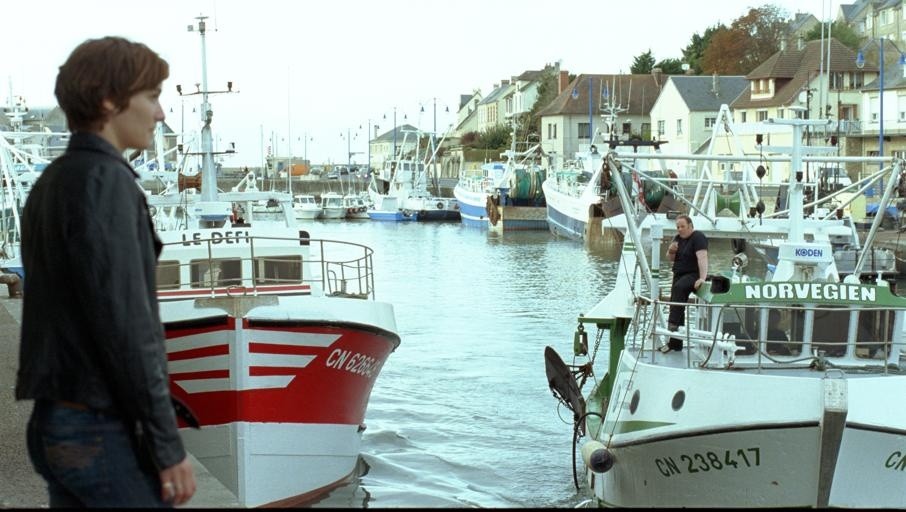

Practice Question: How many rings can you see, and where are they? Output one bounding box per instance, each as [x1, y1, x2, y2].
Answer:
[161, 483, 173, 489]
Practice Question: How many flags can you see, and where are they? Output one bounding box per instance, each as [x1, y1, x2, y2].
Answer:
[629, 170, 645, 210]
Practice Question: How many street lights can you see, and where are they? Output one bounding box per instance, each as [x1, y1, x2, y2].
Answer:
[854, 37, 905, 199]
[340, 96, 449, 177]
[571, 76, 610, 153]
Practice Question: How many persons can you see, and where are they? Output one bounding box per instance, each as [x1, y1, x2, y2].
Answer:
[745, 309, 791, 358]
[11, 34, 198, 509]
[664, 215, 710, 351]
